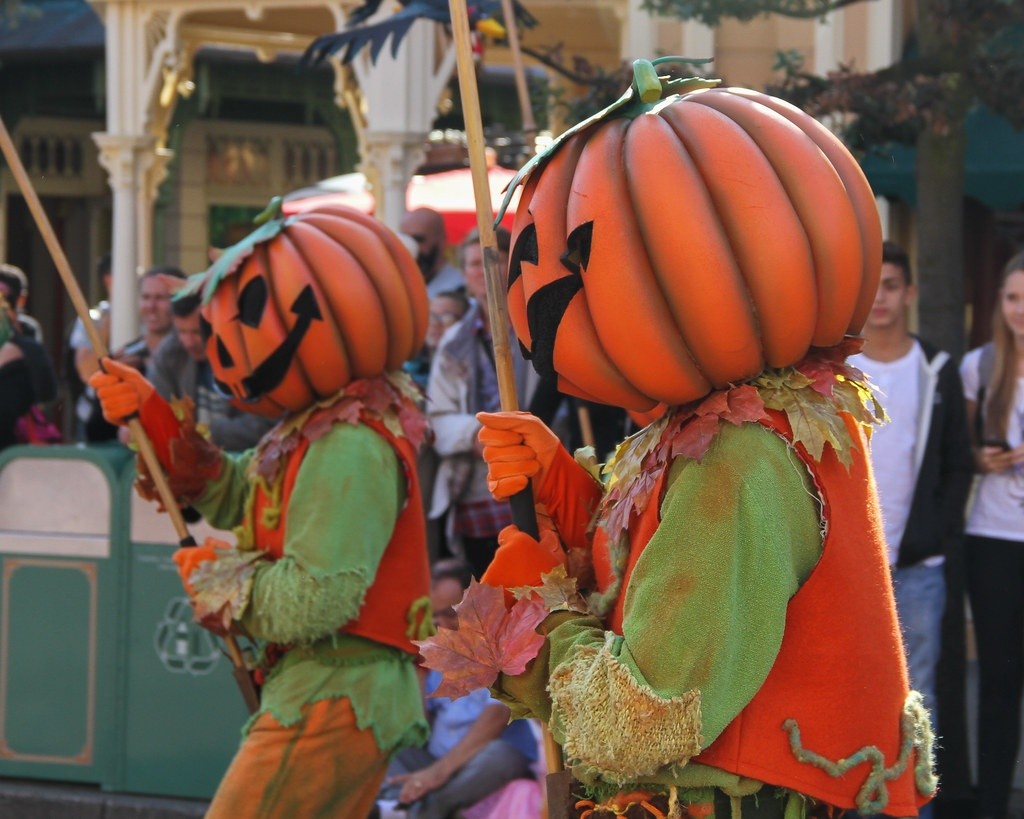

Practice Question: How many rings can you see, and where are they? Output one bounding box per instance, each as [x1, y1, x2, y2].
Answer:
[413, 780, 422, 787]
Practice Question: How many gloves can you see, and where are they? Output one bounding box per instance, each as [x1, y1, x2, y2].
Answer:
[89, 356, 156, 427]
[478, 523, 569, 612]
[172, 537, 231, 596]
[476, 410, 560, 503]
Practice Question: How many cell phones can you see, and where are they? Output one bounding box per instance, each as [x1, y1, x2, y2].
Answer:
[983, 438, 1010, 452]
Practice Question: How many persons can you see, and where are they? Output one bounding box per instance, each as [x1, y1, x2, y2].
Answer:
[381, 208, 568, 819]
[847, 242, 971, 819]
[0, 264, 57, 450]
[88, 198, 437, 819]
[476, 60, 941, 819]
[70, 252, 283, 454]
[960, 250, 1024, 819]
